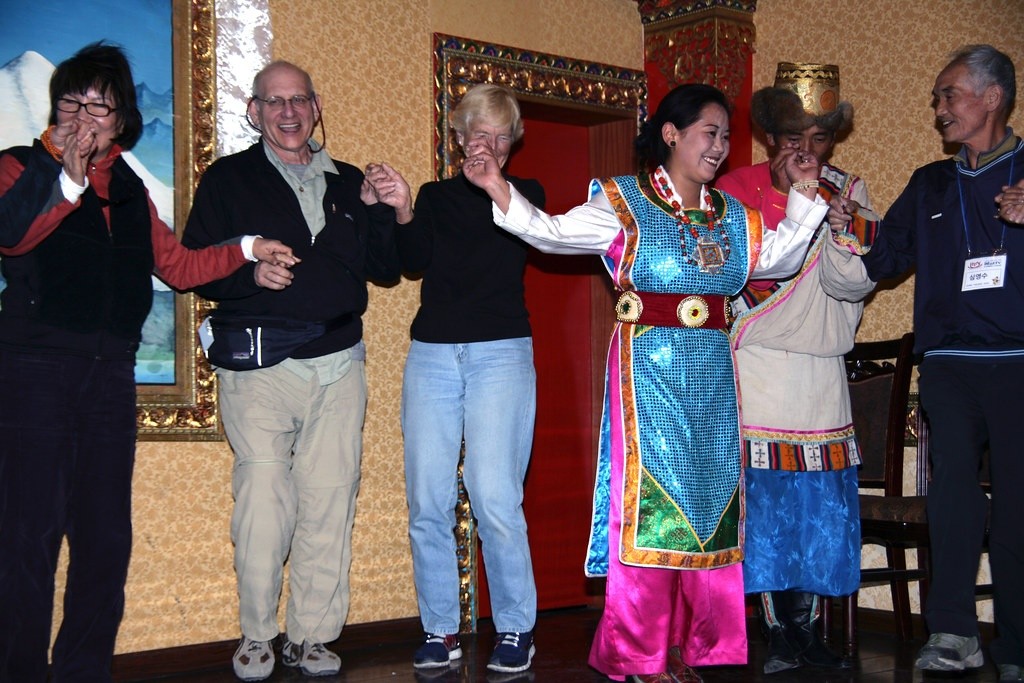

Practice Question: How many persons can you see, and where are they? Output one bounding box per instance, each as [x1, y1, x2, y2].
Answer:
[0, 42, 292, 683]
[714, 60, 879, 683]
[830, 44, 1024, 683]
[365, 83, 547, 672]
[181, 60, 401, 682]
[462, 82, 829, 683]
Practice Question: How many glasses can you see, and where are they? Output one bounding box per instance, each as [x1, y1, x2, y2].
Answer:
[53, 97, 120, 117]
[255, 95, 315, 109]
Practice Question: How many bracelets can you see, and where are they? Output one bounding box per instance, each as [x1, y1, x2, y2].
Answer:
[793, 180, 819, 190]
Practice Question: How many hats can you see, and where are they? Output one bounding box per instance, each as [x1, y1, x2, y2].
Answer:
[751, 59, 853, 139]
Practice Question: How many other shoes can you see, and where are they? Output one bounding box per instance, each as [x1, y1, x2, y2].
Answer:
[663, 650, 703, 683]
[633, 672, 674, 683]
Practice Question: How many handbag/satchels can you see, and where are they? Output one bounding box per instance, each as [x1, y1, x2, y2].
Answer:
[199, 311, 328, 372]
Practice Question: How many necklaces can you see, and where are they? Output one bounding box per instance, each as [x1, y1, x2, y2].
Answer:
[657, 169, 730, 274]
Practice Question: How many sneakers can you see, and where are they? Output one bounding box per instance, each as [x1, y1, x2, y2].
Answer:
[914, 626, 984, 672]
[284, 636, 341, 676]
[232, 629, 274, 682]
[486, 632, 535, 672]
[414, 629, 462, 669]
[997, 664, 1024, 683]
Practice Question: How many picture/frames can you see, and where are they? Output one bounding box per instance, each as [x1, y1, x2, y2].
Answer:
[0, 0, 229, 445]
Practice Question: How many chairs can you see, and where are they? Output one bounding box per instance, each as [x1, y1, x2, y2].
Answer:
[822, 332, 999, 673]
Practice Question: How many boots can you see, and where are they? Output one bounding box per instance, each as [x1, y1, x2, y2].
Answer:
[782, 591, 854, 670]
[760, 591, 804, 673]
